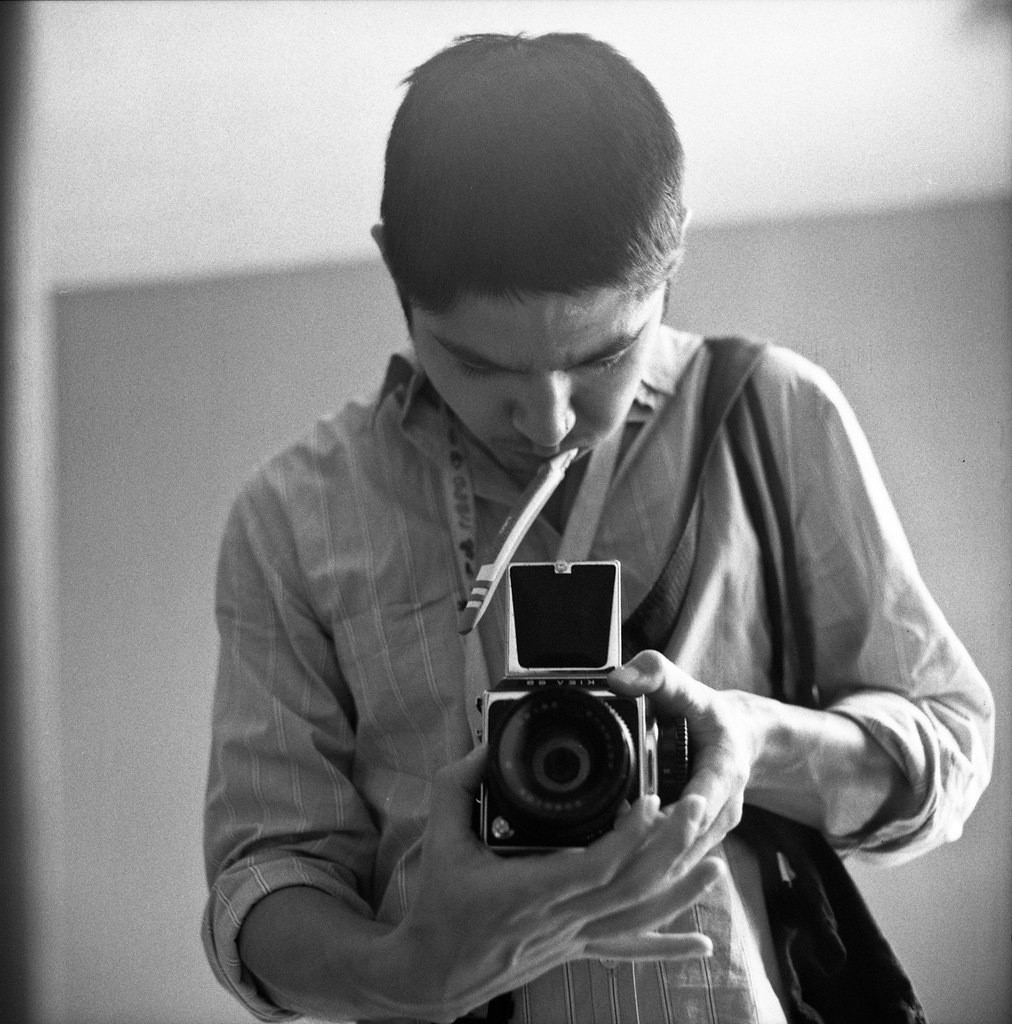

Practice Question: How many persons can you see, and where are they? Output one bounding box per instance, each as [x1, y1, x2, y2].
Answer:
[201, 29, 998, 1023]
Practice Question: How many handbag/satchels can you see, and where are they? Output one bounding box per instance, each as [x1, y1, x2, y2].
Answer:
[731, 801, 929, 1024]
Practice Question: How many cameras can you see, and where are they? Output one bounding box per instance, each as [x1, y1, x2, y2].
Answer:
[475, 558, 690, 860]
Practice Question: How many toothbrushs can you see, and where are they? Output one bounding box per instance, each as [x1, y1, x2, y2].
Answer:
[456, 447, 582, 636]
